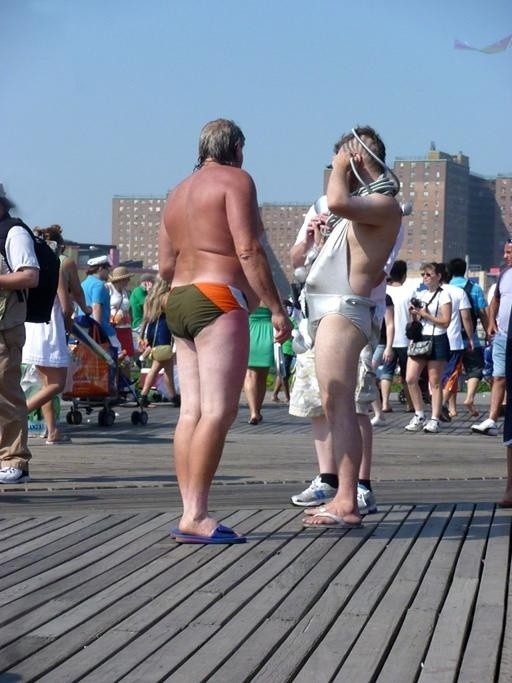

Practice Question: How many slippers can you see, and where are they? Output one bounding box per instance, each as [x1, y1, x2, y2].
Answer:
[46, 434, 72, 444]
[304, 505, 327, 516]
[370, 416, 386, 426]
[175, 524, 247, 544]
[170, 528, 183, 538]
[302, 511, 364, 529]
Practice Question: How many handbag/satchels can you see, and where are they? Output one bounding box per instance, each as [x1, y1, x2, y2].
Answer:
[406, 289, 441, 357]
[109, 287, 123, 325]
[149, 314, 174, 362]
[405, 321, 423, 340]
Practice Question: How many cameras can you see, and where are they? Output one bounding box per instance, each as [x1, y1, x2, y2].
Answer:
[410, 298, 426, 308]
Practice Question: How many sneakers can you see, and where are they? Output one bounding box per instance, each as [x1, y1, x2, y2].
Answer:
[423, 419, 440, 433]
[471, 418, 498, 436]
[356, 489, 377, 515]
[0, 466, 31, 483]
[138, 395, 156, 407]
[439, 406, 451, 423]
[404, 413, 427, 432]
[289, 475, 338, 507]
[172, 394, 180, 406]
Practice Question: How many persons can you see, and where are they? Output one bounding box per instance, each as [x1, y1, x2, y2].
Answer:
[371, 293, 395, 428]
[379, 260, 412, 414]
[497, 307, 512, 507]
[405, 262, 453, 433]
[245, 299, 274, 425]
[447, 259, 489, 416]
[482, 283, 497, 387]
[271, 316, 299, 402]
[470, 238, 512, 435]
[105, 266, 135, 375]
[0, 183, 40, 485]
[300, 125, 404, 531]
[157, 117, 292, 543]
[138, 272, 181, 408]
[76, 255, 121, 356]
[287, 192, 404, 515]
[128, 272, 154, 341]
[22, 225, 73, 445]
[436, 263, 474, 422]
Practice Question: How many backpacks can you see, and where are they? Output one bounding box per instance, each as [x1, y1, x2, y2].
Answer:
[1, 216, 60, 325]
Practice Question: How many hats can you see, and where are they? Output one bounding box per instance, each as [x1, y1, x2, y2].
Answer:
[137, 272, 155, 283]
[107, 266, 135, 282]
[87, 255, 111, 267]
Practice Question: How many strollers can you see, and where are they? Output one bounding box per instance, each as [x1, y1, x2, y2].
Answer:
[65, 308, 148, 427]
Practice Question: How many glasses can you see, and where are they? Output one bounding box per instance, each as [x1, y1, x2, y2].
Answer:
[507, 239, 512, 243]
[420, 272, 434, 277]
